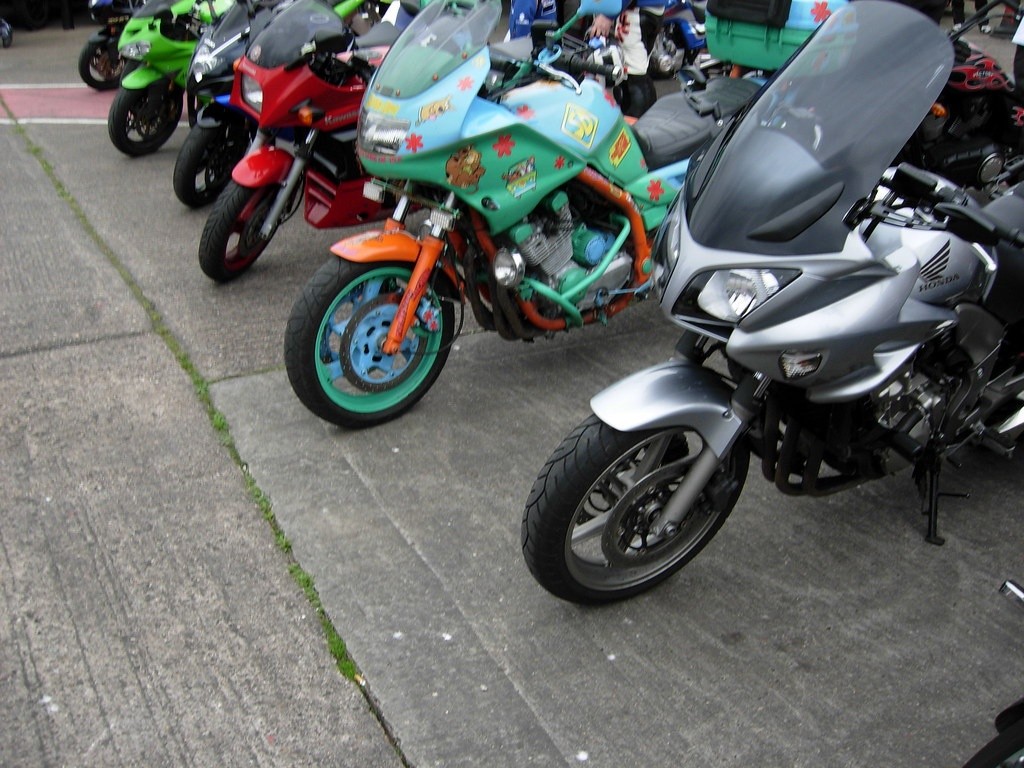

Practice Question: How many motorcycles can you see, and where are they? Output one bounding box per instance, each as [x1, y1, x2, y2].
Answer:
[522, 0, 1024, 768]
[79, 0, 770, 431]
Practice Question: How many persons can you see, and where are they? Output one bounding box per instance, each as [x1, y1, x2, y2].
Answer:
[590, 0, 667, 119]
[951, 0, 992, 34]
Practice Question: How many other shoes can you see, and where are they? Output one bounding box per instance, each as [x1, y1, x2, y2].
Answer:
[948, 22, 964, 39]
[978, 18, 991, 35]
